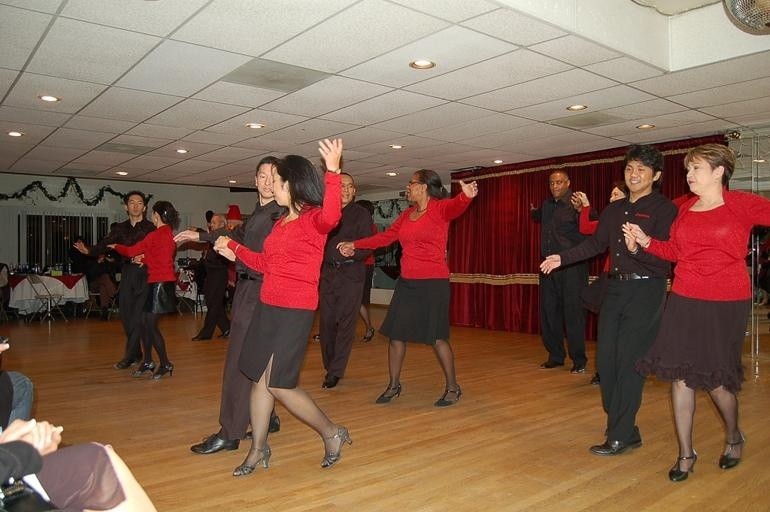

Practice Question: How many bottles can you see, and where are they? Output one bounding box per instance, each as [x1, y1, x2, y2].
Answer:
[10, 261, 72, 276]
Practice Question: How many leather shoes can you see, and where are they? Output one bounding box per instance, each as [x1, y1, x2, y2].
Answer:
[590, 425, 641, 456]
[570, 361, 587, 374]
[192, 334, 212, 342]
[191, 434, 239, 454]
[540, 360, 564, 369]
[218, 329, 229, 340]
[322, 374, 338, 388]
[114, 352, 143, 369]
[246, 417, 280, 438]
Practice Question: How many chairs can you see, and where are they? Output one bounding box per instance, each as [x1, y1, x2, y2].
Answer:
[0, 268, 226, 324]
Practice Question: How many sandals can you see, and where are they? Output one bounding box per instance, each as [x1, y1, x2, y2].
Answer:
[362, 328, 375, 342]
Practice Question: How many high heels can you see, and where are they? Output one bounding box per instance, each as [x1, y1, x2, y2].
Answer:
[435, 385, 461, 406]
[151, 362, 173, 379]
[233, 442, 271, 476]
[376, 384, 401, 403]
[321, 425, 352, 468]
[668, 451, 697, 481]
[130, 361, 156, 378]
[718, 438, 743, 469]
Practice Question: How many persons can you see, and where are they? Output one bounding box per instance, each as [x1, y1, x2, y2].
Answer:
[337, 169, 478, 406]
[531, 170, 592, 373]
[71, 191, 181, 380]
[172, 136, 378, 477]
[1, 343, 155, 512]
[543, 144, 770, 481]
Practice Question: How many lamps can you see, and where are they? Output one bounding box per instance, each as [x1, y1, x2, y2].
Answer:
[723, 0, 770, 36]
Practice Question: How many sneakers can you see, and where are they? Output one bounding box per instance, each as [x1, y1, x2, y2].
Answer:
[591, 372, 601, 385]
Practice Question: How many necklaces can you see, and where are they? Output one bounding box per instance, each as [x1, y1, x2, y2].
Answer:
[417, 207, 427, 213]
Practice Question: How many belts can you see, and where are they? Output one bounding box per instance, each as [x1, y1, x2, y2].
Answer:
[328, 259, 354, 265]
[238, 273, 254, 280]
[612, 272, 649, 280]
